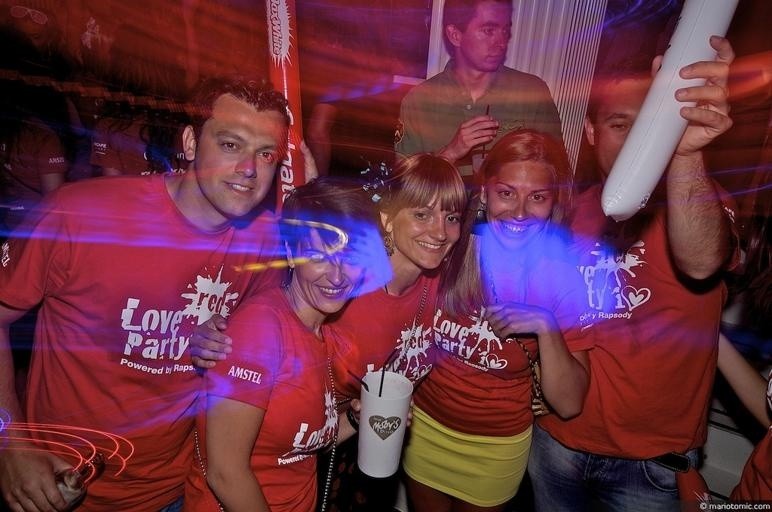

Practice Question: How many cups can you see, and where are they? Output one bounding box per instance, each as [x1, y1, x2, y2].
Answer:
[357, 371, 415, 480]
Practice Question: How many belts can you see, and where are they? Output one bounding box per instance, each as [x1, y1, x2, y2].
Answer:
[652, 451, 691, 474]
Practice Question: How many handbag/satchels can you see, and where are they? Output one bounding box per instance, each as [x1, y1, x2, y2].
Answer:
[530, 359, 551, 420]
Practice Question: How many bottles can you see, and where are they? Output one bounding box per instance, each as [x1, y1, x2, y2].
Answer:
[55, 451, 106, 508]
